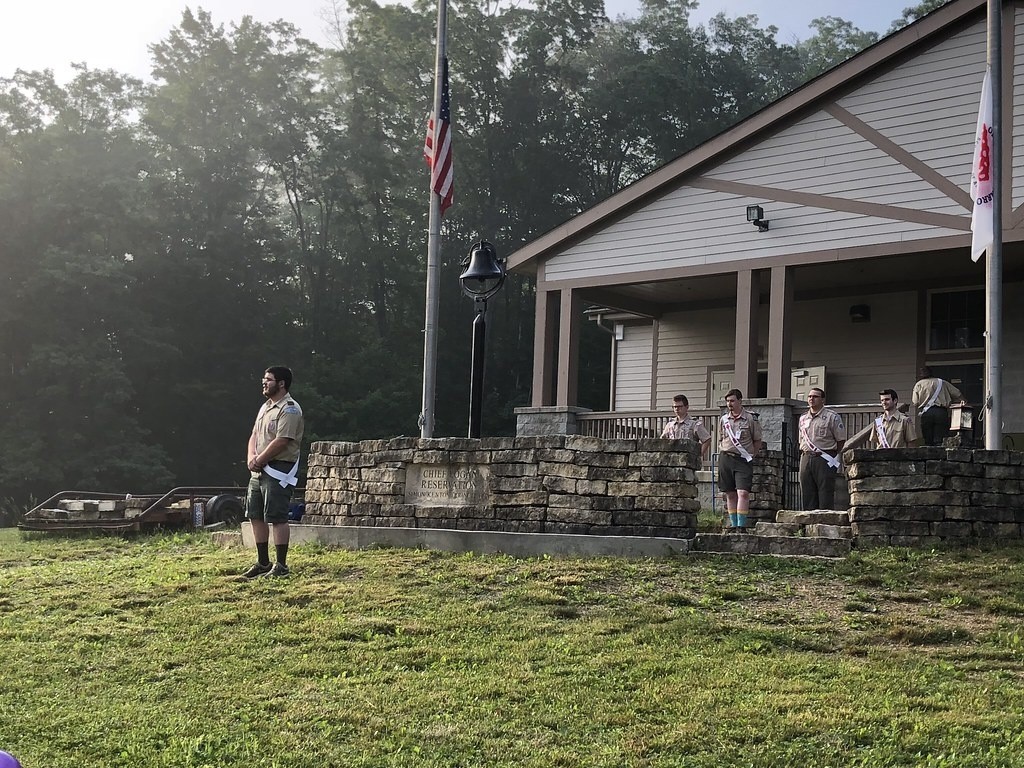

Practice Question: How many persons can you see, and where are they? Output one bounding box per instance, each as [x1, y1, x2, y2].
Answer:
[241, 366, 305, 581]
[798, 387, 846, 511]
[912, 366, 968, 447]
[718, 389, 761, 527]
[869, 389, 917, 448]
[659, 395, 711, 457]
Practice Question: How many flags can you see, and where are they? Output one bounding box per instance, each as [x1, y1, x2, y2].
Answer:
[424, 55, 454, 215]
[970, 69, 994, 262]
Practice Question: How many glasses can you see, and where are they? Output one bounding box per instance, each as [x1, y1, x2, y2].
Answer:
[808, 394, 821, 398]
[261, 376, 280, 384]
[671, 406, 684, 409]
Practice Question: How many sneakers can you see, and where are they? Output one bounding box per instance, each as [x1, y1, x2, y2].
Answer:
[264, 562, 290, 580]
[242, 561, 273, 580]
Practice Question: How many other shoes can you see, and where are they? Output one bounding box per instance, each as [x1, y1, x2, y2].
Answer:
[728, 527, 737, 533]
[736, 526, 748, 533]
